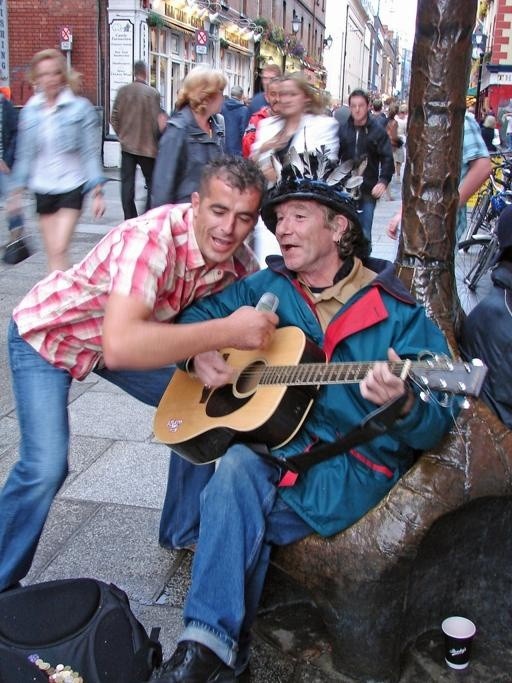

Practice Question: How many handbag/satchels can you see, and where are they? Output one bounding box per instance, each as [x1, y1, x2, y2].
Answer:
[4, 236, 31, 265]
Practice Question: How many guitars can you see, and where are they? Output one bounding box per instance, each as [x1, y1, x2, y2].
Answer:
[151, 323, 489, 467]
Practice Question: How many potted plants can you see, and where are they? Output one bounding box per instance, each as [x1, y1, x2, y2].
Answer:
[219, 38, 227, 58]
[145, 13, 163, 29]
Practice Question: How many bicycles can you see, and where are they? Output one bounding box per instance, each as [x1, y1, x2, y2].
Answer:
[456, 152, 512, 317]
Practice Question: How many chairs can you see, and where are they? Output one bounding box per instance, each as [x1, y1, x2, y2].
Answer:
[187, 0, 511, 683]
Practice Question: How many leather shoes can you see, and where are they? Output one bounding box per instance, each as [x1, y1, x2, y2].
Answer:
[149, 638, 252, 683]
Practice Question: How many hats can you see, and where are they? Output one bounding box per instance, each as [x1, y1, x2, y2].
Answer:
[258, 123, 366, 248]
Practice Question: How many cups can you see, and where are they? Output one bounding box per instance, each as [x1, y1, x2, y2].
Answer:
[441, 615, 478, 671]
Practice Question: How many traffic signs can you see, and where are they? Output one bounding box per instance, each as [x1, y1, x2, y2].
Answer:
[196, 30, 207, 45]
[60, 27, 72, 41]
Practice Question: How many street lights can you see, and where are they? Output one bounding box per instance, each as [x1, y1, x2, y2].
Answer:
[473, 33, 487, 121]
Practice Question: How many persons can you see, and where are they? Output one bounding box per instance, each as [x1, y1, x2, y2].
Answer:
[0, 155, 279, 594]
[479, 104, 498, 155]
[385, 86, 492, 259]
[242, 74, 283, 158]
[361, 92, 412, 186]
[112, 63, 167, 220]
[338, 91, 394, 253]
[153, 63, 226, 209]
[245, 67, 284, 126]
[0, 92, 30, 265]
[459, 205, 512, 429]
[250, 71, 343, 191]
[149, 143, 463, 683]
[216, 86, 250, 158]
[11, 49, 107, 276]
